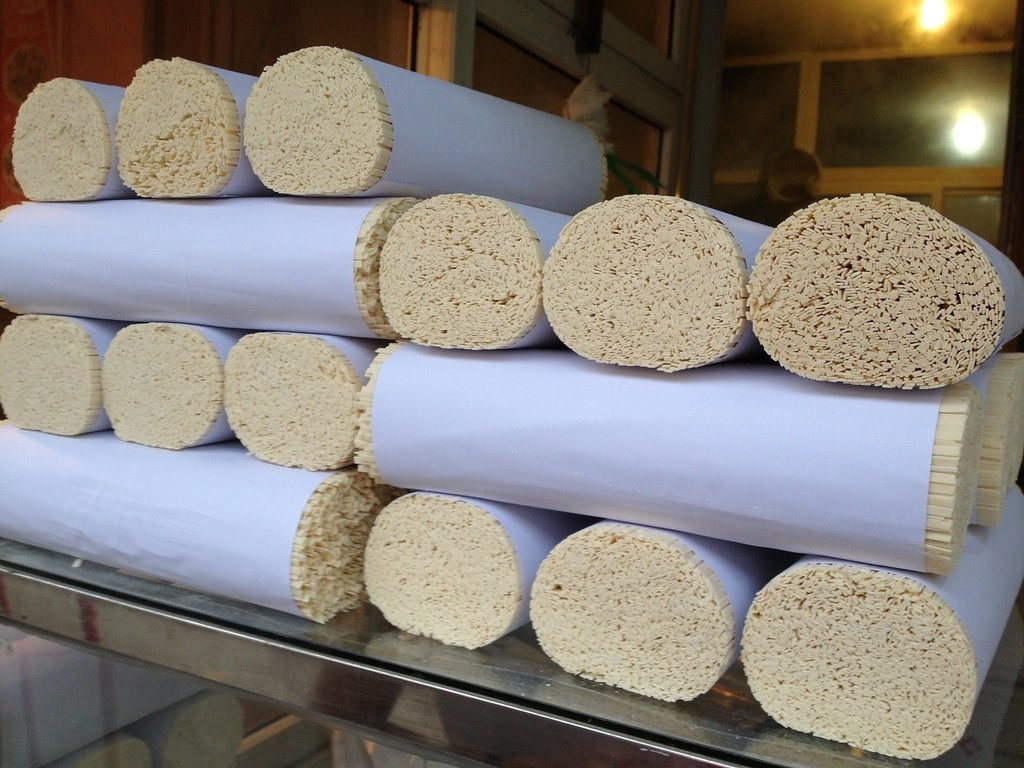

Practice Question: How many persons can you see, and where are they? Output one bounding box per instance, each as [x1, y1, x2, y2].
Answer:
[740, 149, 822, 228]
